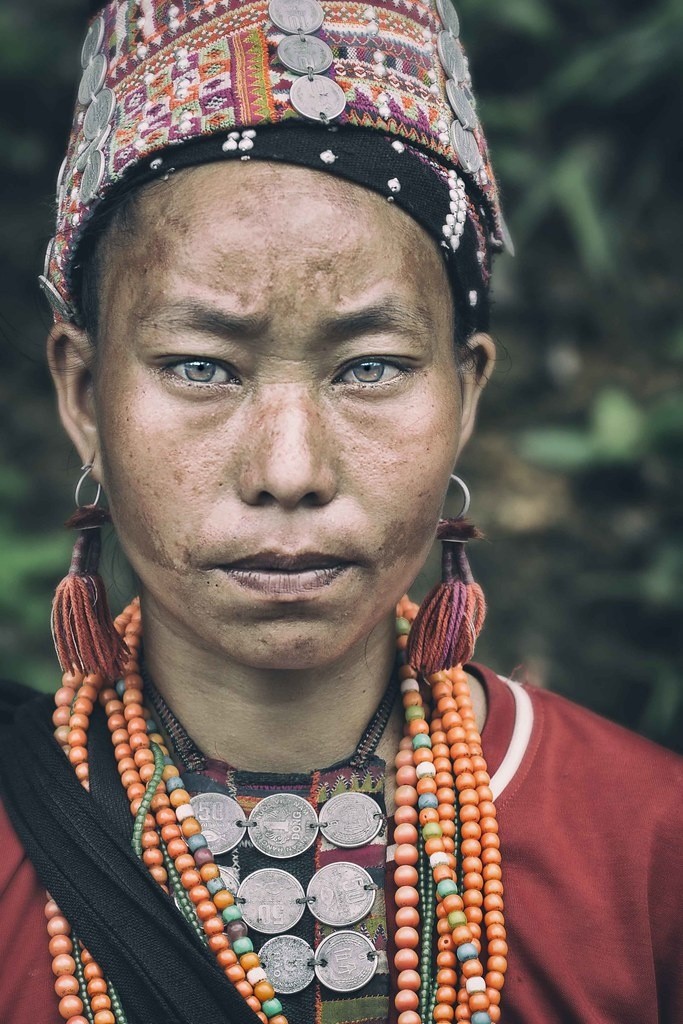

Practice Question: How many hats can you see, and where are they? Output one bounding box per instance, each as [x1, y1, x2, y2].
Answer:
[36, 0, 514, 329]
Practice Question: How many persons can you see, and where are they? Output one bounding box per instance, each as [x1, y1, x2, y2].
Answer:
[0, 0, 683, 1024]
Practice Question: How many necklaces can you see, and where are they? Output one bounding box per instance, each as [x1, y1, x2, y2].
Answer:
[46, 593, 507, 1024]
